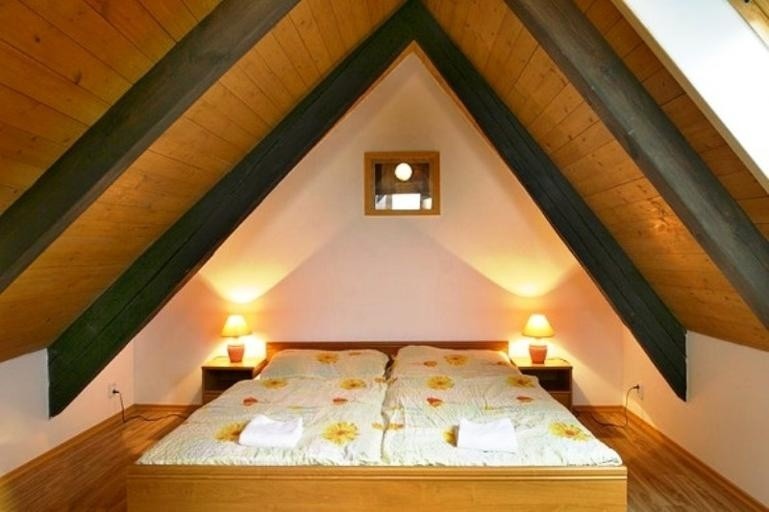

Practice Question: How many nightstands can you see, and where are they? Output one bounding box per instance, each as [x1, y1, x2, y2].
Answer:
[511, 356, 573, 415]
[200, 355, 266, 406]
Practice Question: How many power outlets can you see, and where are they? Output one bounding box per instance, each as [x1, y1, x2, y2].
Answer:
[107, 384, 120, 399]
[633, 382, 644, 400]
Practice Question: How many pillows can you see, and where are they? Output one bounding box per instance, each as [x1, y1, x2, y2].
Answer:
[262, 345, 519, 382]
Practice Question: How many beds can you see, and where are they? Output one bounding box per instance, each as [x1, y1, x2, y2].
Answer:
[125, 340, 629, 512]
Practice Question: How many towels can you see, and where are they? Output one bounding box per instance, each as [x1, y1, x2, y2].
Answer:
[238, 412, 304, 448]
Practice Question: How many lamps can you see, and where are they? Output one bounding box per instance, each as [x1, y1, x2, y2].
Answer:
[521, 312, 556, 364]
[220, 314, 254, 363]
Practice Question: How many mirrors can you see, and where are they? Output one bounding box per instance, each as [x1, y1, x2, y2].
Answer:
[363, 150, 442, 217]
